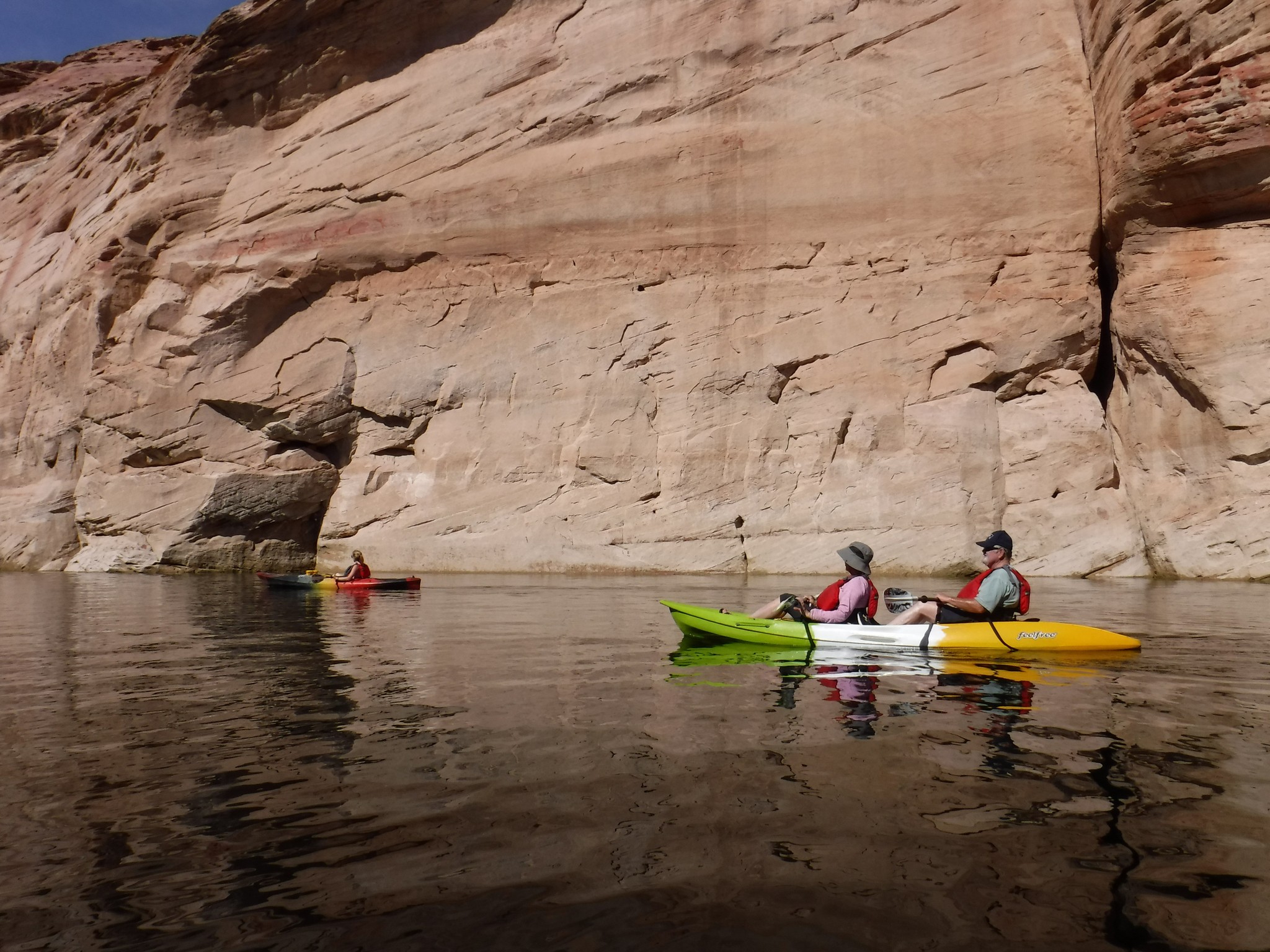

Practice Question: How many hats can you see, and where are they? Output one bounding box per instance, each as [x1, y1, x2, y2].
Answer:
[837, 542, 874, 575]
[976, 530, 1013, 551]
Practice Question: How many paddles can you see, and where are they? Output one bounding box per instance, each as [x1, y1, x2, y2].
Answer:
[884, 586, 939, 614]
[773, 592, 825, 612]
[311, 573, 348, 585]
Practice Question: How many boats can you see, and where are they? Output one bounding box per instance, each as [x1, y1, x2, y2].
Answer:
[657, 599, 1142, 653]
[256, 571, 422, 590]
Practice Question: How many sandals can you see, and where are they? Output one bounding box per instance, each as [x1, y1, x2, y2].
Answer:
[719, 608, 731, 615]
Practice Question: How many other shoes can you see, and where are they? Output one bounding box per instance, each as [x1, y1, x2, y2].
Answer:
[867, 613, 880, 625]
[857, 612, 872, 626]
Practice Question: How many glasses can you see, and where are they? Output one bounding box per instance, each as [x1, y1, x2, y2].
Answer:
[984, 546, 999, 552]
[351, 555, 354, 558]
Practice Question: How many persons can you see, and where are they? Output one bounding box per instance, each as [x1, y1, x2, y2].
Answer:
[857, 530, 1031, 625]
[325, 549, 370, 582]
[719, 541, 879, 625]
